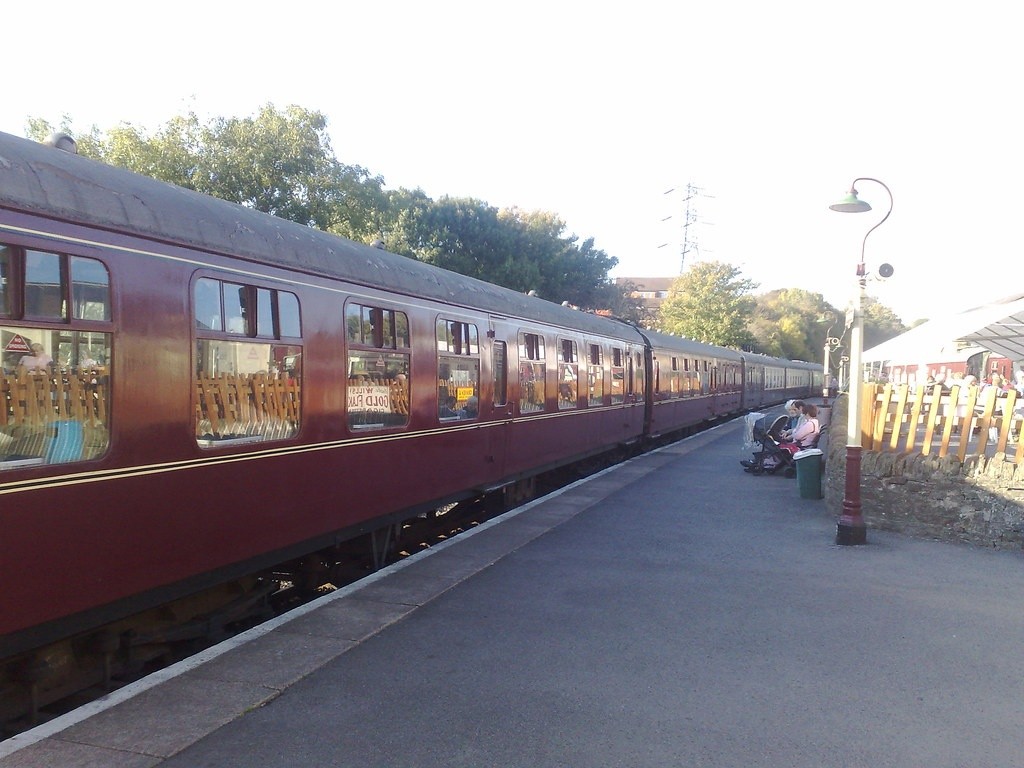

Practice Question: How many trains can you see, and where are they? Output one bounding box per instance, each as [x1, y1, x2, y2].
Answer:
[0, 130, 835, 736]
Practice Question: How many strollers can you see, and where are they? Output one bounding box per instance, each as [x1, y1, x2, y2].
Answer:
[741, 412, 828, 479]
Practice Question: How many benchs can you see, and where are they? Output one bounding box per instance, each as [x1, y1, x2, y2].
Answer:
[786, 423, 828, 466]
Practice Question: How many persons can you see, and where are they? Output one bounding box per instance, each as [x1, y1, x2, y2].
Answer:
[15, 343, 53, 375]
[739, 398, 820, 473]
[355, 372, 407, 394]
[832, 378, 839, 397]
[924, 371, 1024, 443]
[79, 348, 98, 383]
[441, 396, 478, 419]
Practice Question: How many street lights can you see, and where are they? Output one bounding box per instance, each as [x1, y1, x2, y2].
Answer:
[827, 176, 894, 547]
[817, 310, 838, 409]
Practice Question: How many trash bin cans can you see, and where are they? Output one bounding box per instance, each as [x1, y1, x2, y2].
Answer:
[792, 449, 824, 499]
